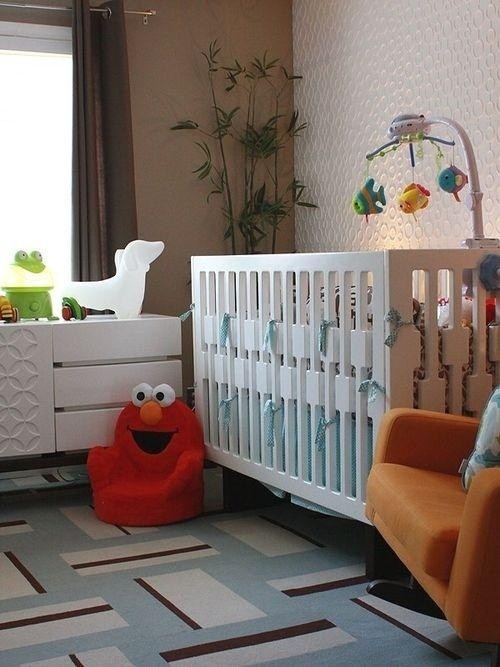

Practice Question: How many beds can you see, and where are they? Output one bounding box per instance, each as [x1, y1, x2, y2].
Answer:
[188, 251, 497, 585]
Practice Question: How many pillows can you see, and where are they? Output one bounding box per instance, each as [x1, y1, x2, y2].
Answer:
[455, 391, 498, 492]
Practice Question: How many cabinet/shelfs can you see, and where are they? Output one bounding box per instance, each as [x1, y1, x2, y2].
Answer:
[2, 312, 186, 499]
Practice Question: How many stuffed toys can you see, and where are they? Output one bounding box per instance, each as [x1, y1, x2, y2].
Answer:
[438, 166, 468, 202]
[399, 183, 430, 214]
[352, 178, 386, 215]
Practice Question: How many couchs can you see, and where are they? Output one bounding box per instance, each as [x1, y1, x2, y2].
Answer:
[362, 390, 500, 643]
